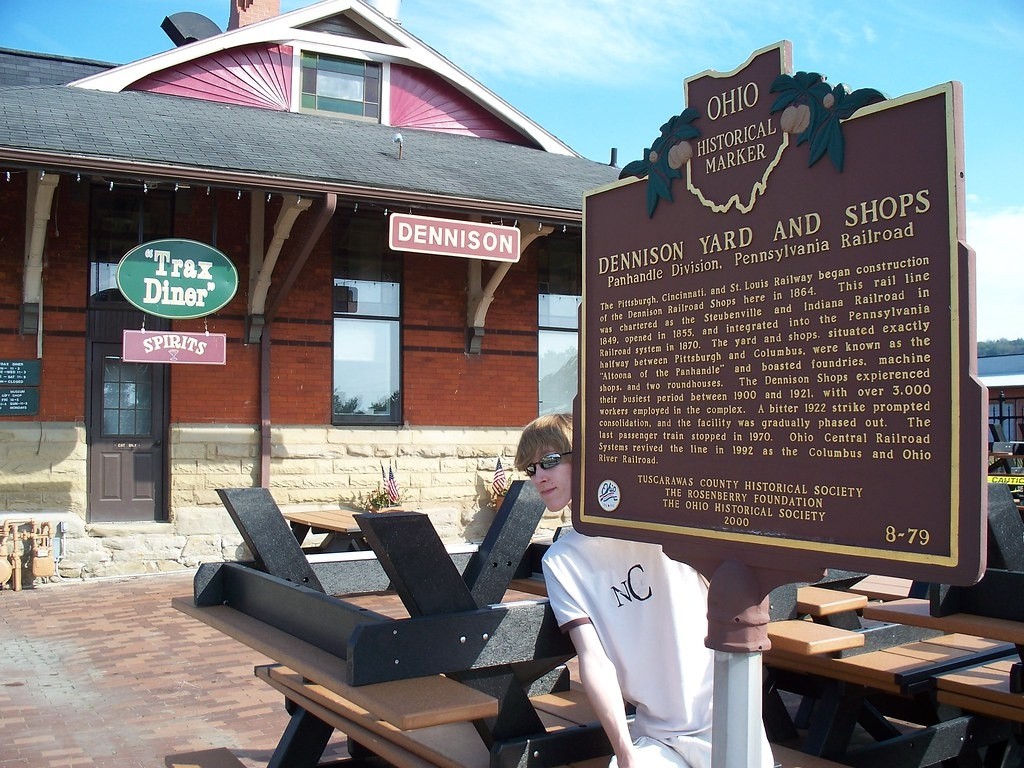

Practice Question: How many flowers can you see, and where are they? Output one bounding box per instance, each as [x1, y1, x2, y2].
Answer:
[360, 487, 396, 509]
[491, 472, 513, 502]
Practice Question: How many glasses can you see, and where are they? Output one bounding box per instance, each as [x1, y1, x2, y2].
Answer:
[524, 453, 571, 476]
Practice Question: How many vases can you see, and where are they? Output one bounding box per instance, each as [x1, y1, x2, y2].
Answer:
[494, 494, 505, 512]
[376, 507, 403, 514]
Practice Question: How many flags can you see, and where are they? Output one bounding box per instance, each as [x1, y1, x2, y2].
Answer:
[382, 468, 389, 493]
[493, 459, 506, 495]
[388, 466, 399, 501]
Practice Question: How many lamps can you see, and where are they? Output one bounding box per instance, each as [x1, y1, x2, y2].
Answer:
[392, 133, 404, 160]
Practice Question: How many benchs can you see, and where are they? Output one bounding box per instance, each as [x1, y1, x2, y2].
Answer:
[989, 452, 1013, 457]
[171, 596, 498, 729]
[796, 587, 868, 617]
[863, 598, 1024, 646]
[764, 619, 865, 653]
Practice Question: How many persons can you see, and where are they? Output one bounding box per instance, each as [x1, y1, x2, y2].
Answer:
[514, 412, 773, 768]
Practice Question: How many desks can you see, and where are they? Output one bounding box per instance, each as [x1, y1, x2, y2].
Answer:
[215, 423, 1024, 768]
